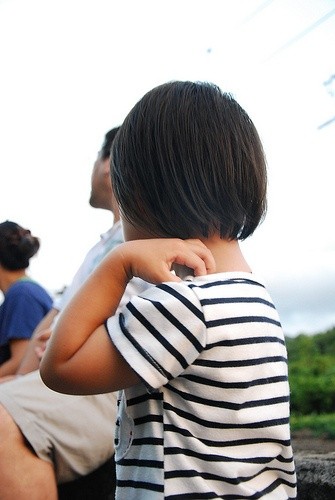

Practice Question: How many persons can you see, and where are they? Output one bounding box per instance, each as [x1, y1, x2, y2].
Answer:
[1, 220, 53, 378]
[39, 80, 297, 500]
[1, 126, 120, 499]
